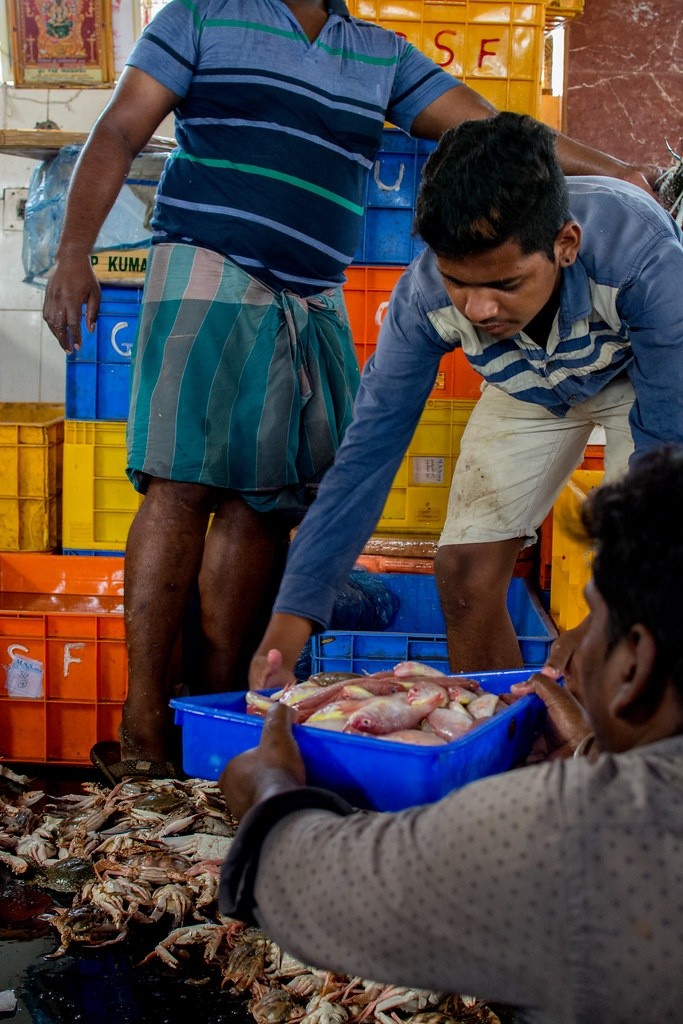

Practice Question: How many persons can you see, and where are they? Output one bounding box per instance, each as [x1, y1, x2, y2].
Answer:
[215, 446, 683, 1023]
[249, 113, 683, 696]
[43, 0, 664, 787]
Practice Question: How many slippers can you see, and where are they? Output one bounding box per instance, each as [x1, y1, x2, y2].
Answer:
[90, 740, 176, 791]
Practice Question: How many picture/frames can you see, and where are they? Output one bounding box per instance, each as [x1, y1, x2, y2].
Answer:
[6, 0, 114, 89]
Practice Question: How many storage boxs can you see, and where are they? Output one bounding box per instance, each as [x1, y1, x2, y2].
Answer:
[0, 0, 587, 813]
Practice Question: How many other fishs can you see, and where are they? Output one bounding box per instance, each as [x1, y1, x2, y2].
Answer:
[246, 661, 511, 748]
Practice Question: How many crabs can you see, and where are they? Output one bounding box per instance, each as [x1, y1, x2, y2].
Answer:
[0, 778, 502, 1023]
